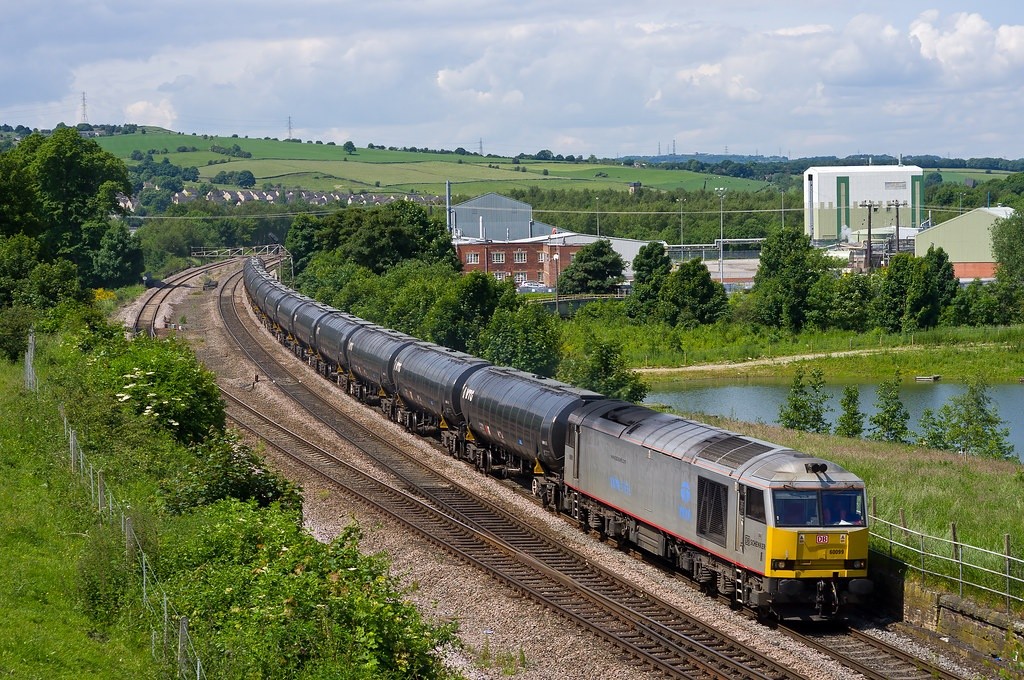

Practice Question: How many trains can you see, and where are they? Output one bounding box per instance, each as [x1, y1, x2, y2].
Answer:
[242, 253, 874, 625]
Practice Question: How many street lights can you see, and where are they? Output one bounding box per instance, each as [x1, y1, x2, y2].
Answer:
[885, 199, 909, 253]
[676, 198, 687, 263]
[553, 253, 559, 312]
[859, 199, 883, 267]
[715, 187, 728, 288]
